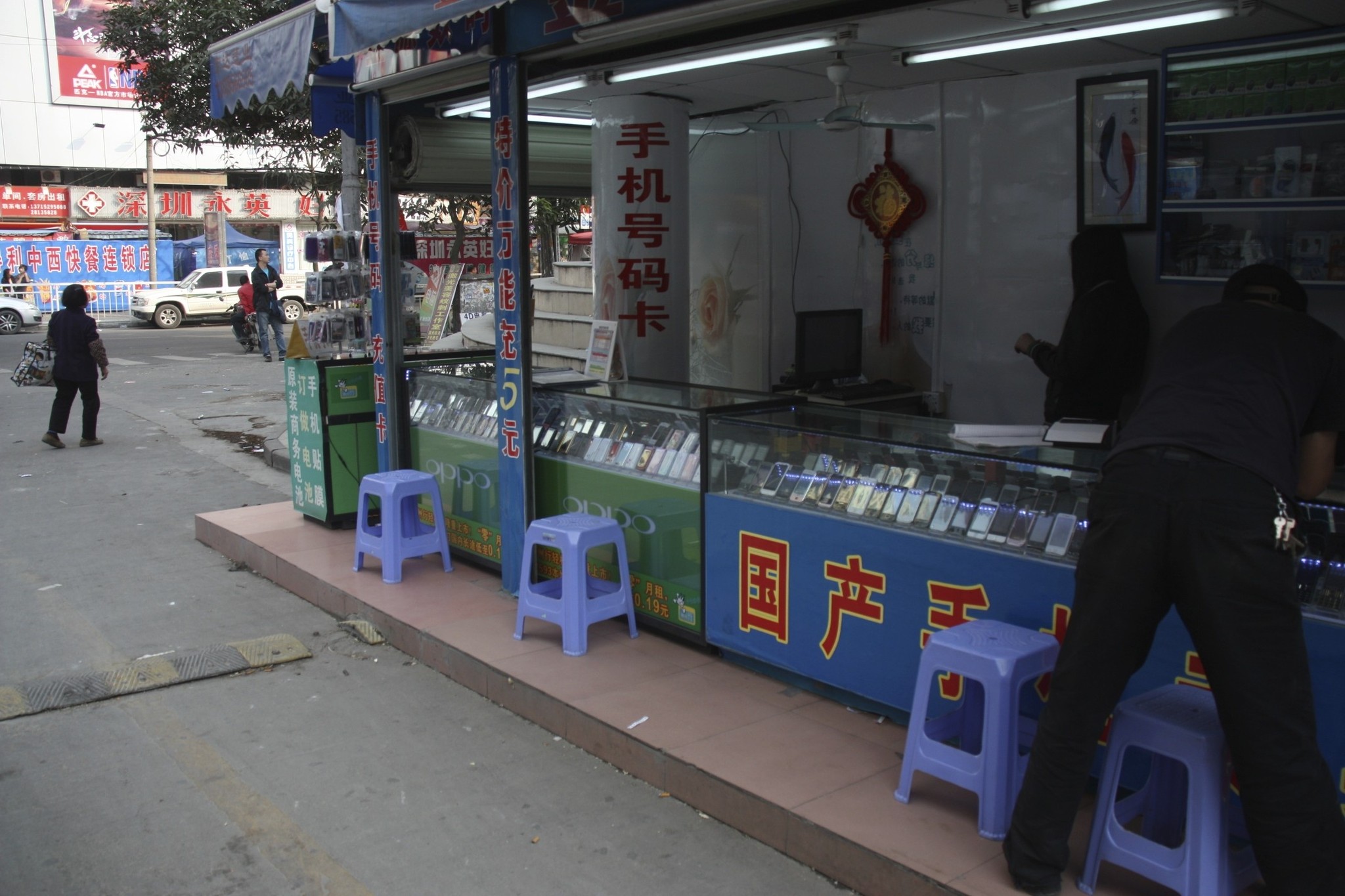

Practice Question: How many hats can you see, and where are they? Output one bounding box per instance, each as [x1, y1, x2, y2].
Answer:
[1220, 264, 1307, 312]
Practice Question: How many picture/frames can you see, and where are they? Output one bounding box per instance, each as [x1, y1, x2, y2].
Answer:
[1077, 67, 1156, 233]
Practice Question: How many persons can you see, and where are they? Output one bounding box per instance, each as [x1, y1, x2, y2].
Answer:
[324, 261, 344, 273]
[1003, 259, 1345, 896]
[40, 285, 108, 447]
[250, 248, 287, 362]
[465, 265, 478, 275]
[231, 276, 256, 343]
[1, 264, 31, 300]
[1013, 227, 1151, 419]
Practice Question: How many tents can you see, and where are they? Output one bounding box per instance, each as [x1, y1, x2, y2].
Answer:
[171, 221, 280, 276]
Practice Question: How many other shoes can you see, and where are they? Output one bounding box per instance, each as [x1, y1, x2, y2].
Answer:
[279, 358, 285, 361]
[1004, 829, 1064, 894]
[236, 337, 248, 342]
[43, 436, 66, 448]
[80, 438, 102, 447]
[265, 355, 272, 362]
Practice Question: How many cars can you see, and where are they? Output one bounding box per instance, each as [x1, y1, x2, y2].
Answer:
[0, 295, 42, 335]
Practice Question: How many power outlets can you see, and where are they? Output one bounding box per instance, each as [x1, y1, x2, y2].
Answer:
[921, 391, 944, 415]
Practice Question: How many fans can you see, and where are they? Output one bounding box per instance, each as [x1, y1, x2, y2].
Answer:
[741, 51, 935, 132]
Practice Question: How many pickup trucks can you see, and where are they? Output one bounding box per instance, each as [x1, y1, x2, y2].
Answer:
[129, 267, 317, 330]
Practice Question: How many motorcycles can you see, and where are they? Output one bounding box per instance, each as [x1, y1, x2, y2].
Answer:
[227, 303, 273, 355]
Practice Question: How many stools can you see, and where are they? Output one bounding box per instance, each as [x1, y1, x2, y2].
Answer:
[1076, 681, 1267, 896]
[612, 502, 701, 589]
[896, 619, 1060, 840]
[512, 512, 639, 655]
[353, 468, 454, 583]
[452, 458, 498, 528]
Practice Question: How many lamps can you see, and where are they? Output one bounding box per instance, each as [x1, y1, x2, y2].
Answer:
[436, 74, 601, 126]
[600, 23, 860, 85]
[1004, 0, 1116, 19]
[890, 0, 1248, 68]
[82, 123, 105, 138]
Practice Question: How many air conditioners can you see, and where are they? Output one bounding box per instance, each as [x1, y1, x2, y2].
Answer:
[41, 170, 61, 184]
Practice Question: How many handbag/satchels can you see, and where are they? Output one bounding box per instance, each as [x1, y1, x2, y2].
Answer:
[10, 339, 56, 387]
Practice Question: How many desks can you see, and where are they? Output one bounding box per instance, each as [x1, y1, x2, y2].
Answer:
[776, 384, 920, 413]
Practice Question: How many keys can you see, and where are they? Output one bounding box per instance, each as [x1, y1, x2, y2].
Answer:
[1272, 516, 1304, 551]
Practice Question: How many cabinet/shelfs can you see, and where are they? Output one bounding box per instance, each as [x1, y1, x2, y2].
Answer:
[1155, 31, 1345, 297]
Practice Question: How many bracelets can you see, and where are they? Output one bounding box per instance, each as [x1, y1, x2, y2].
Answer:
[1027, 339, 1041, 355]
